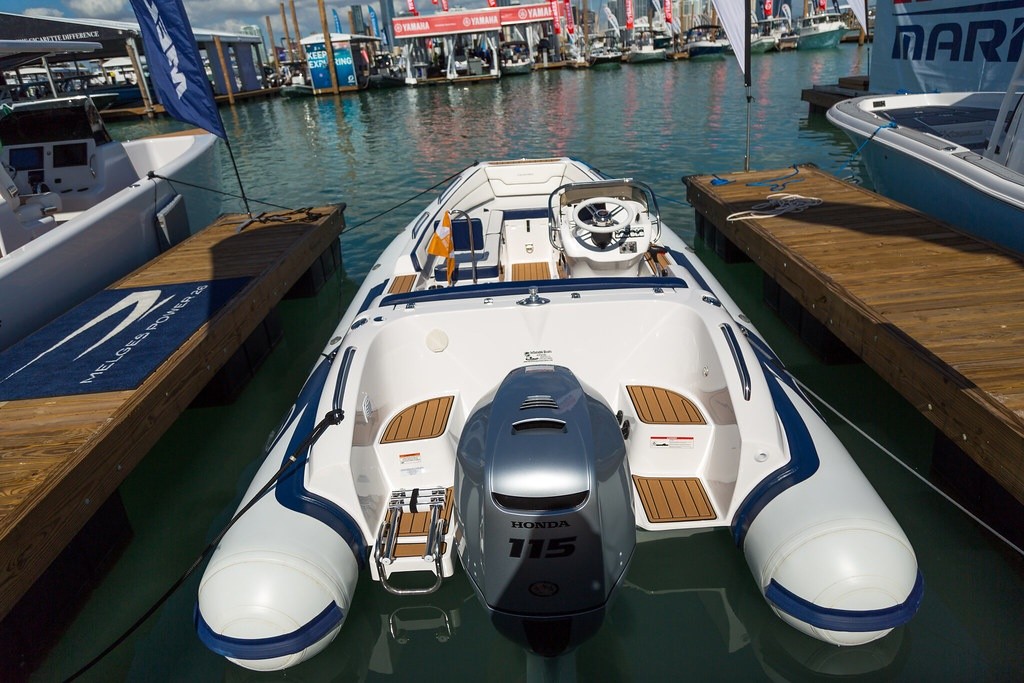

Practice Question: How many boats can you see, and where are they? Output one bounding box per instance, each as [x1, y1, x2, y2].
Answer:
[1, 0, 873, 98]
[192, 156, 926, 676]
[0, 35, 220, 349]
[825, 39, 1024, 263]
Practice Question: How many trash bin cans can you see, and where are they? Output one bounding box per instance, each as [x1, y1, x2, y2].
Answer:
[469, 58, 482, 75]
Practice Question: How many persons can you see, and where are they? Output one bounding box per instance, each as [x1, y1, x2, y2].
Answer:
[265, 53, 406, 88]
[431, 20, 723, 76]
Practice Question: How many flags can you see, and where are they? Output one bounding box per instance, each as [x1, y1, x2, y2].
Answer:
[426, 212, 455, 284]
